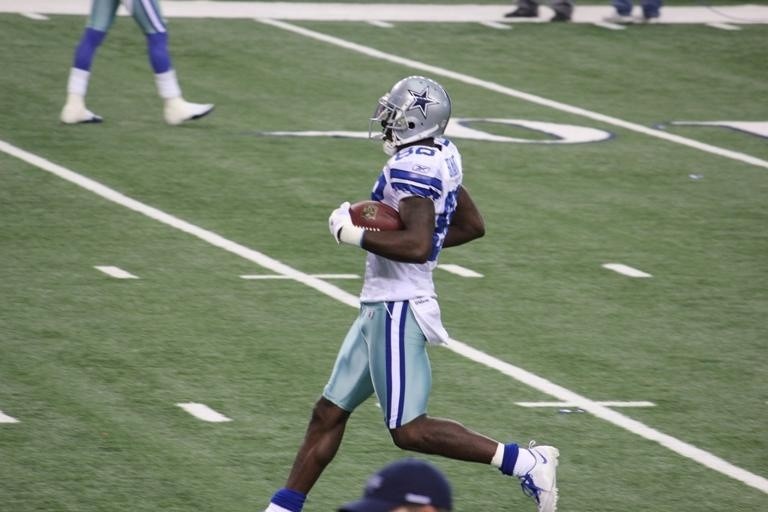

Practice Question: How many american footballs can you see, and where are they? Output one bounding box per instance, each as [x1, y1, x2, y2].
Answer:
[349, 201, 404, 231]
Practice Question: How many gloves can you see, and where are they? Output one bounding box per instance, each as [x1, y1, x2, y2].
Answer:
[328, 200, 353, 245]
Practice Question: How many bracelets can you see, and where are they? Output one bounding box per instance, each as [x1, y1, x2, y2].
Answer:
[340, 224, 364, 247]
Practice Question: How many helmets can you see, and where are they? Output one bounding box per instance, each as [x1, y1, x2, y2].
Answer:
[378, 74, 452, 147]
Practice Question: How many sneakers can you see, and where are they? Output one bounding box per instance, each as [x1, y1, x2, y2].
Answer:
[163, 96, 215, 127]
[518, 439, 560, 512]
[639, 16, 661, 26]
[59, 102, 104, 125]
[607, 13, 634, 25]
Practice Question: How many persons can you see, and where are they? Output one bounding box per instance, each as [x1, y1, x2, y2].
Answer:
[504, 0, 573, 23]
[602, 0, 662, 24]
[263, 76, 559, 512]
[60, 0, 215, 125]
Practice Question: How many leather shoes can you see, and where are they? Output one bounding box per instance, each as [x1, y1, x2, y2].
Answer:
[505, 7, 539, 17]
[550, 12, 571, 23]
